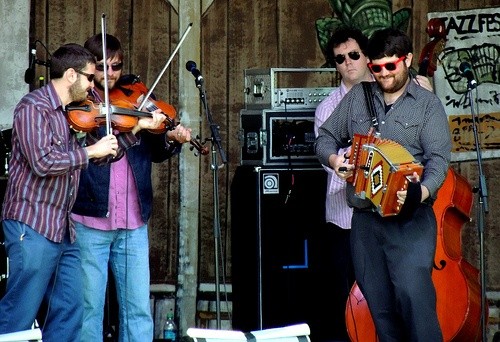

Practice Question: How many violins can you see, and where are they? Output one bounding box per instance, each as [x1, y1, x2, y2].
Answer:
[108, 74, 210, 157]
[65, 100, 172, 133]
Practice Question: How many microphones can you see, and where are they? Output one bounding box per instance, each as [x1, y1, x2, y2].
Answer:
[24, 44, 37, 84]
[186, 61, 204, 83]
[459, 62, 476, 88]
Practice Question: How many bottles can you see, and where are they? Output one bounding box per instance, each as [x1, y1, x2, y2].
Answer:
[163, 312, 177, 342]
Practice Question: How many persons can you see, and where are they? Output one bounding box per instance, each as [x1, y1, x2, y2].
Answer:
[0, 43, 118, 335]
[314, 26, 453, 342]
[314, 27, 435, 342]
[65, 33, 193, 342]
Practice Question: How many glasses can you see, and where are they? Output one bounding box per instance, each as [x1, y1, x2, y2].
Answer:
[367, 55, 406, 74]
[333, 51, 360, 65]
[74, 69, 95, 82]
[96, 60, 124, 71]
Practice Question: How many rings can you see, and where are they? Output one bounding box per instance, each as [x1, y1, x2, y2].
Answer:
[178, 133, 181, 137]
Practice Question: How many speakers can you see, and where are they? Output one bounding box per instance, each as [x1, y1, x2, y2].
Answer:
[228, 166, 357, 342]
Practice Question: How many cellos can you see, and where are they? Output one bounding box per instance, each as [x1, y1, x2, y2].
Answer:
[345, 165, 488, 342]
